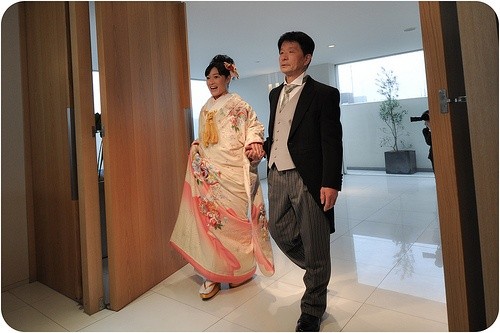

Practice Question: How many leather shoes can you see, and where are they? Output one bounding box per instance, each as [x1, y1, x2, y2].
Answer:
[295, 312, 322, 332]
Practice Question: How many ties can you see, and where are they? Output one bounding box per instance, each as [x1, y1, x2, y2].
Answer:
[280, 76, 307, 112]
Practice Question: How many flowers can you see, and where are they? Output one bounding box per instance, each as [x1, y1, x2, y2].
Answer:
[224, 62, 240, 79]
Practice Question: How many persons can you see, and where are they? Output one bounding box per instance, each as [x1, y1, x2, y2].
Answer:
[168, 54, 265, 298]
[245, 30, 344, 332]
[419, 110, 435, 173]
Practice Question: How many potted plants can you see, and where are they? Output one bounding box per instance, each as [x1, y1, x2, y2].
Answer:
[375, 68, 416, 174]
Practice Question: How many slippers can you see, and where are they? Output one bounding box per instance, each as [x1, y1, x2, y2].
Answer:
[199, 279, 221, 298]
[228, 276, 252, 288]
